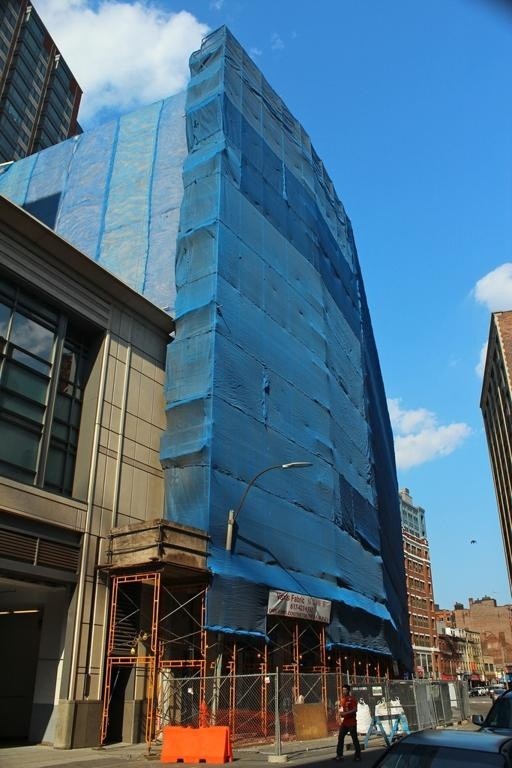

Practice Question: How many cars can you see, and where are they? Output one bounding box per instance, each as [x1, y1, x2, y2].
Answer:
[472, 687, 512, 734]
[469, 682, 507, 703]
[370, 726, 512, 768]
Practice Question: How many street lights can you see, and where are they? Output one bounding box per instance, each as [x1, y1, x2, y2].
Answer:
[207, 459, 316, 727]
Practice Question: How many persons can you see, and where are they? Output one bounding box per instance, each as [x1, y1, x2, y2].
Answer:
[332, 684, 362, 762]
[291, 686, 304, 705]
[489, 692, 496, 703]
